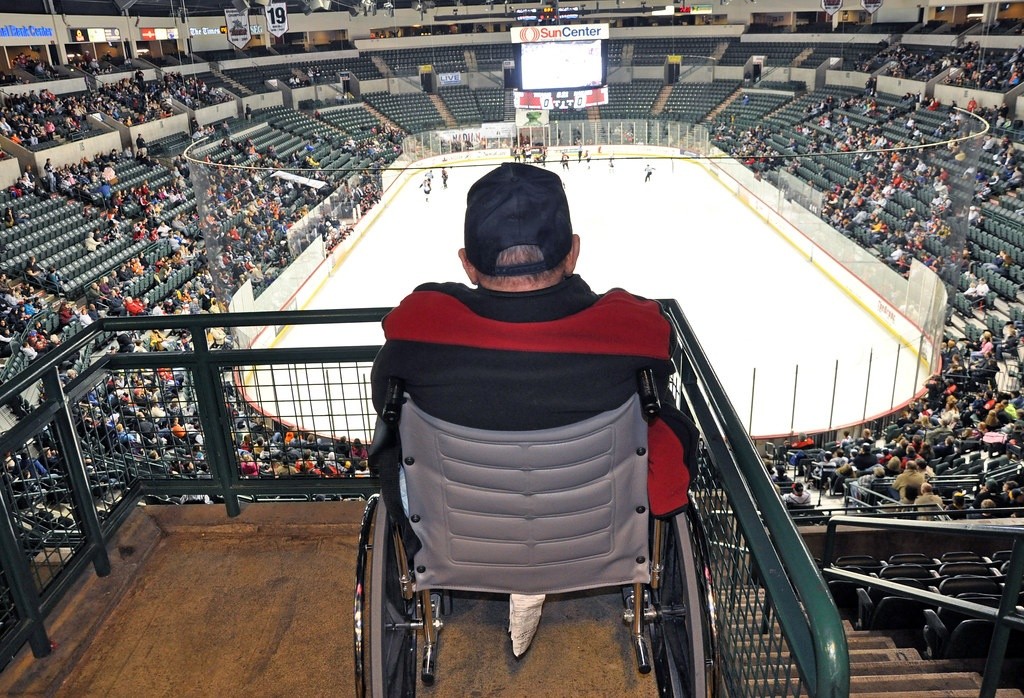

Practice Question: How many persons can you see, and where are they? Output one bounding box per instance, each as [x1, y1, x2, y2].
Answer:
[0, 51, 405, 479]
[514, 132, 614, 169]
[644, 165, 656, 182]
[371, 29, 399, 38]
[382, 163, 701, 520]
[710, 39, 1024, 521]
[421, 168, 448, 201]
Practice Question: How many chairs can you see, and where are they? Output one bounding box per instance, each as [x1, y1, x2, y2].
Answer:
[1016, 606, 1024, 619]
[868, 565, 929, 579]
[1000, 583, 1024, 608]
[938, 593, 1002, 648]
[928, 562, 991, 578]
[857, 577, 926, 620]
[923, 609, 1024, 660]
[927, 577, 997, 614]
[0, 21, 1024, 527]
[932, 551, 980, 564]
[988, 560, 1024, 583]
[856, 587, 939, 631]
[880, 554, 930, 566]
[829, 556, 883, 569]
[982, 549, 1013, 563]
[824, 567, 865, 581]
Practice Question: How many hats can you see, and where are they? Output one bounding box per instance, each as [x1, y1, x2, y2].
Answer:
[273, 432, 282, 440]
[464, 163, 573, 276]
[285, 432, 294, 441]
[327, 452, 335, 461]
[791, 483, 803, 492]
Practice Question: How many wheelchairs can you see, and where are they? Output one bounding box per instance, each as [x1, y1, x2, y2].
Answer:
[353, 368, 719, 698]
